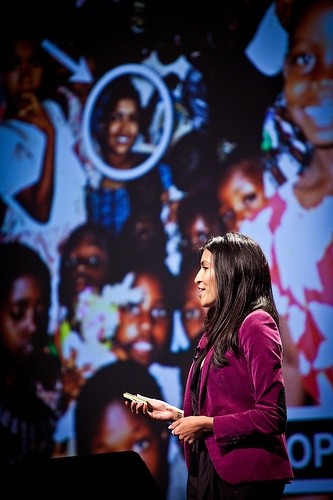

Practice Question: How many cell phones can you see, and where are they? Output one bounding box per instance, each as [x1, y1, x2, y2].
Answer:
[123, 392, 153, 412]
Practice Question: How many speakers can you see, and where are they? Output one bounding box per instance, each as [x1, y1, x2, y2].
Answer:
[0, 451, 163, 500]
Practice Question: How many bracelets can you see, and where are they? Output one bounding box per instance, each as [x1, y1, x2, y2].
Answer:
[176, 410, 183, 420]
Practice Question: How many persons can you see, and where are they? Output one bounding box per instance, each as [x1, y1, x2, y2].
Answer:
[0, 0, 333, 500]
[125, 232, 295, 500]
